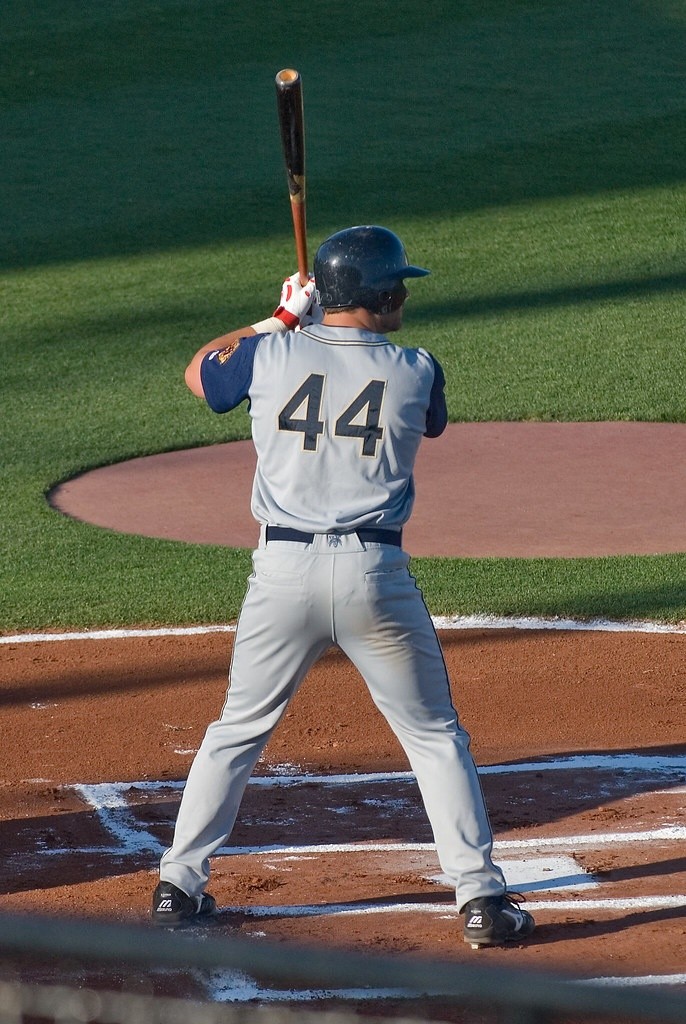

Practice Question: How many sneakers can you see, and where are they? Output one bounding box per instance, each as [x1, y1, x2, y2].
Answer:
[151, 881, 216, 929]
[463, 891, 535, 944]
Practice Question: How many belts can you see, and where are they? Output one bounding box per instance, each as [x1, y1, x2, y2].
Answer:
[266, 526, 401, 547]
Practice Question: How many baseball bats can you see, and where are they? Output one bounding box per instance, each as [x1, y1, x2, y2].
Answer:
[274, 67, 310, 281]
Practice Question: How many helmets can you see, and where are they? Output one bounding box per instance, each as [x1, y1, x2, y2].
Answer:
[314, 225, 430, 315]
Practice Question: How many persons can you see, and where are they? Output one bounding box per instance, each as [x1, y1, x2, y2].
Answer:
[152, 224, 535, 948]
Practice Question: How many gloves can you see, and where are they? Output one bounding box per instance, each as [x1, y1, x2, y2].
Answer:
[273, 271, 316, 329]
